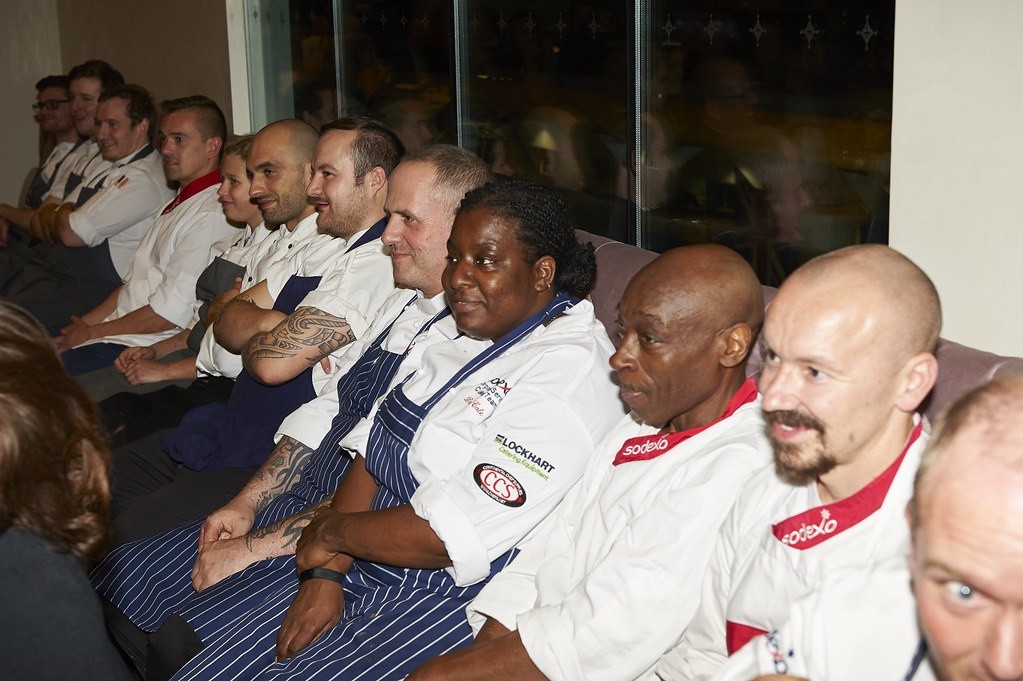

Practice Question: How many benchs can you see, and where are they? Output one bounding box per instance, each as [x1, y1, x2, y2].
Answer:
[565, 226, 1023, 427]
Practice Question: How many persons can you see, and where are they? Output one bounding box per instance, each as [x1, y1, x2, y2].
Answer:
[0, 61, 1023, 681]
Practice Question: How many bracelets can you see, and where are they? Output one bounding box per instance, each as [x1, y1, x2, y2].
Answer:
[299, 567, 345, 588]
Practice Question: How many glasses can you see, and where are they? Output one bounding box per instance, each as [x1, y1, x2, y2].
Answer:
[32, 100, 70, 114]
